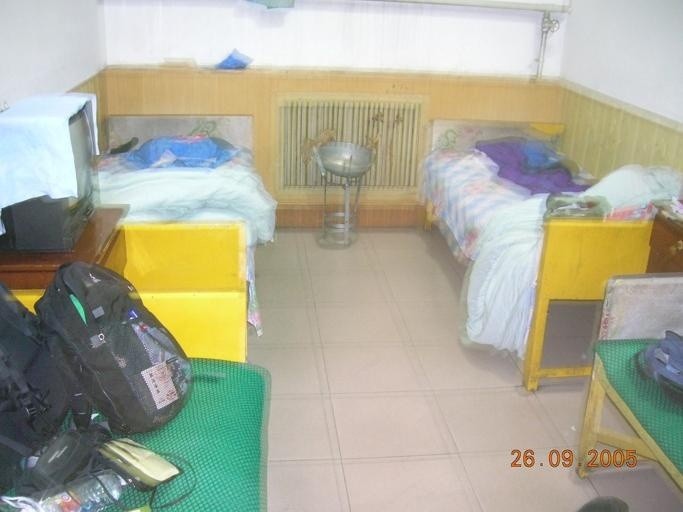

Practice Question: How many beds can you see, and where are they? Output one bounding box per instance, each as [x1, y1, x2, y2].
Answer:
[90, 117, 267, 220]
[419, 120, 658, 395]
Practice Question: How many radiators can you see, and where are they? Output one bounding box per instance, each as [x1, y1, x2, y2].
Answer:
[274, 92, 422, 199]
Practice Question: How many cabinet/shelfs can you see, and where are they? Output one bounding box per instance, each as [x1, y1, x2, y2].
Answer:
[0, 202, 129, 291]
[647, 196, 683, 272]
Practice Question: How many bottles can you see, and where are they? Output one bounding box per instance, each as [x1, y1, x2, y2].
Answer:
[29, 470, 127, 512]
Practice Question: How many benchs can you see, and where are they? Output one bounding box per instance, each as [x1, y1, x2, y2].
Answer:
[575, 272, 682, 501]
[1, 338, 269, 512]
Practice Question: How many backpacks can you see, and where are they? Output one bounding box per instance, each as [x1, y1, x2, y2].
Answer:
[0, 279, 92, 495]
[33, 261, 193, 434]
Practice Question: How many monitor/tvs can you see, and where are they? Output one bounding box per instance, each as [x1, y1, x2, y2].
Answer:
[0, 106, 95, 251]
